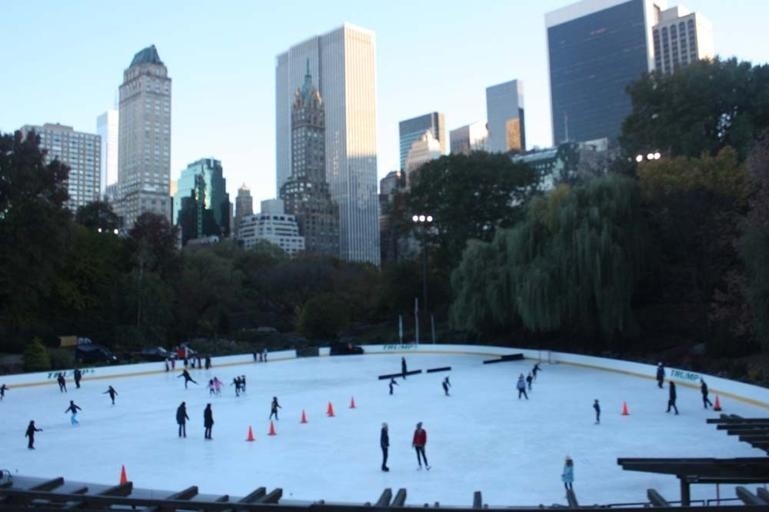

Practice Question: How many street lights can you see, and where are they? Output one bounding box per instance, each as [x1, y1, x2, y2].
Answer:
[405, 212, 435, 346]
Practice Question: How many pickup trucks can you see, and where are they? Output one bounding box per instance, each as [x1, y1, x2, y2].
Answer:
[123, 344, 179, 365]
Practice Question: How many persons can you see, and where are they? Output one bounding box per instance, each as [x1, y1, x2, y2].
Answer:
[65, 401, 82, 425]
[401, 356, 407, 379]
[412, 422, 431, 471]
[269, 396, 282, 420]
[516, 361, 543, 399]
[0, 384, 9, 400]
[102, 385, 118, 405]
[442, 376, 452, 396]
[388, 378, 398, 394]
[176, 402, 189, 438]
[657, 361, 713, 413]
[560, 455, 574, 492]
[57, 373, 68, 392]
[25, 420, 43, 449]
[204, 403, 214, 440]
[166, 342, 268, 397]
[380, 421, 390, 471]
[74, 366, 82, 388]
[592, 399, 601, 424]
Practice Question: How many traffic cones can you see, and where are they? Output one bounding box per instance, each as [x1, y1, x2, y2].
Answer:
[326, 401, 334, 414]
[244, 426, 256, 442]
[299, 409, 308, 424]
[267, 420, 278, 437]
[119, 463, 128, 485]
[348, 397, 357, 409]
[714, 395, 723, 410]
[327, 406, 336, 417]
[620, 401, 631, 416]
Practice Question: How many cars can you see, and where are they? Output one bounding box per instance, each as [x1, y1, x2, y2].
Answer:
[76, 345, 121, 366]
